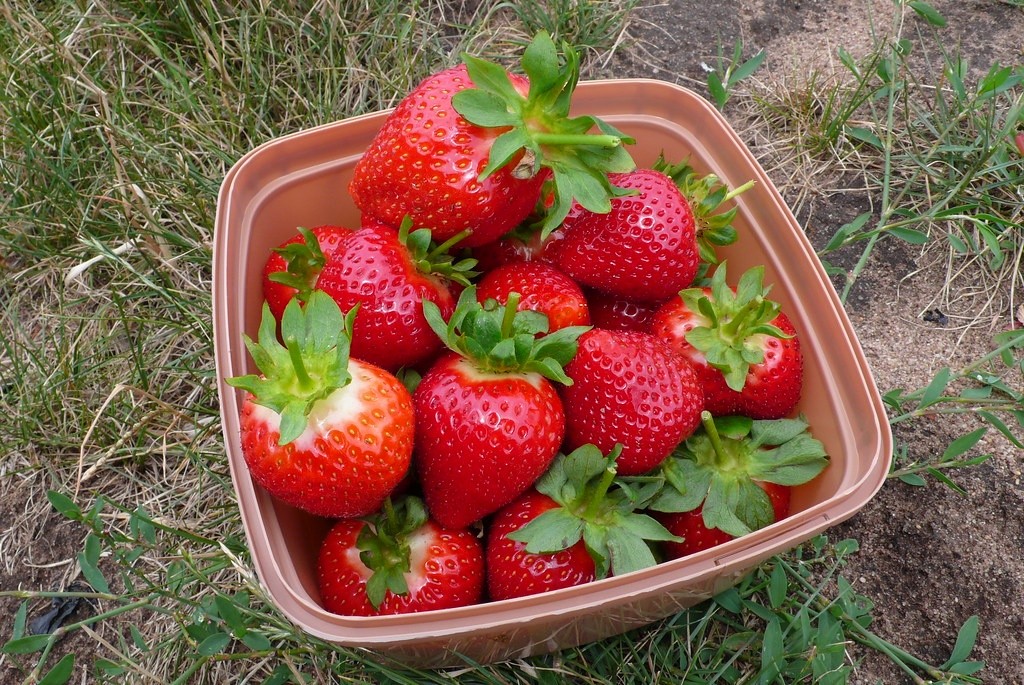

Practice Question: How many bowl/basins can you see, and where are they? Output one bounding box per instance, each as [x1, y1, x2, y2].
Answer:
[211, 79, 894, 669]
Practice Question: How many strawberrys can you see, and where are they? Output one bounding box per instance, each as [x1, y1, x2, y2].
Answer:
[221, 31, 833, 618]
